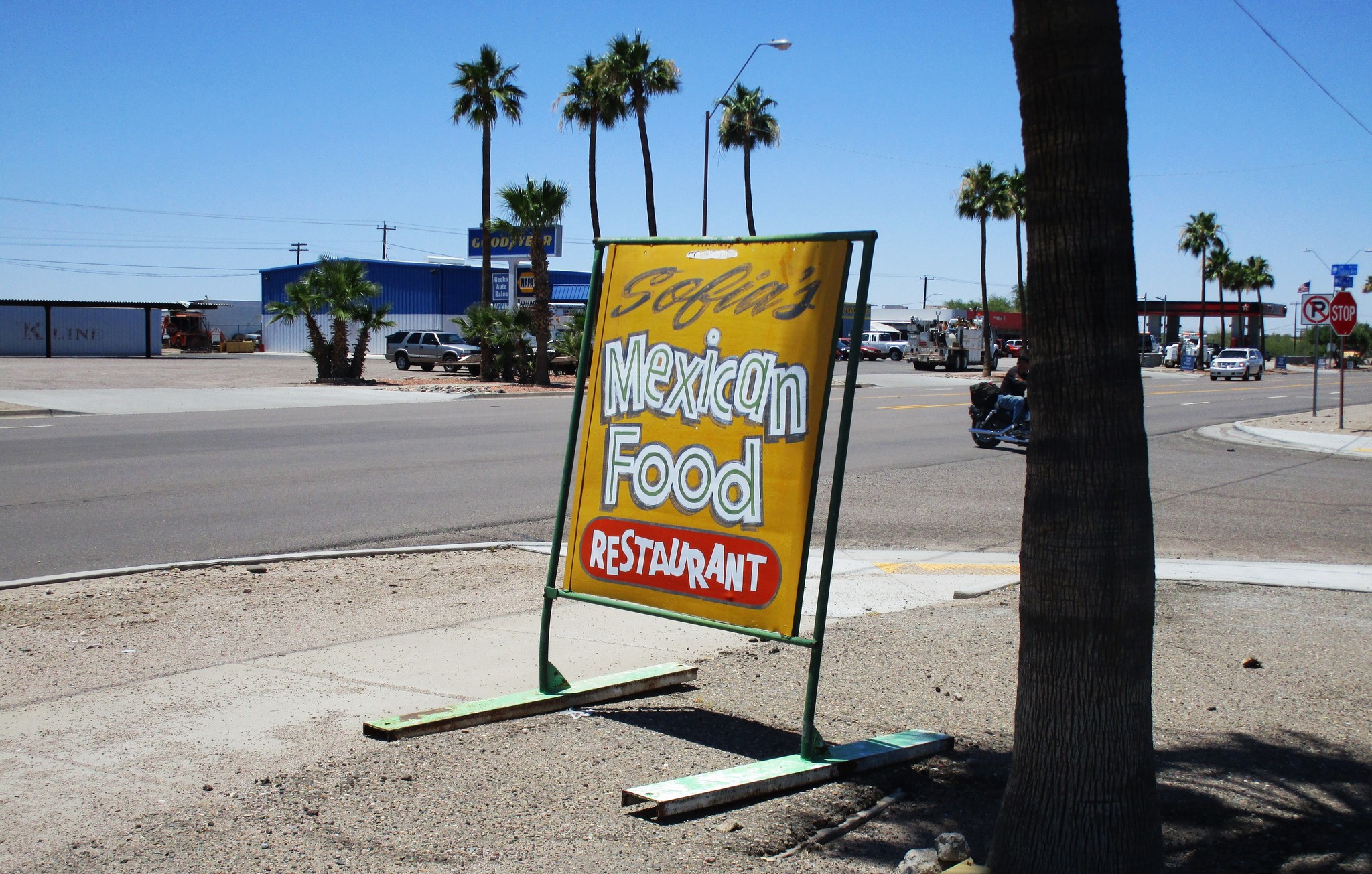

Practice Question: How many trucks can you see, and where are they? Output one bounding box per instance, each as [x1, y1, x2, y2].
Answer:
[546, 302, 587, 316]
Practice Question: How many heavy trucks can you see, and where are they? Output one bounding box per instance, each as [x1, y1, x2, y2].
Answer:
[903, 314, 999, 371]
[1165, 332, 1213, 369]
[993, 339, 1009, 357]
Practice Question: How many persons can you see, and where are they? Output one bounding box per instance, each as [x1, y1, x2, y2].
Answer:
[997, 350, 1029, 436]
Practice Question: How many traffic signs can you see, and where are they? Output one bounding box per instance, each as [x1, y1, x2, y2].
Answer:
[1330, 263, 1359, 288]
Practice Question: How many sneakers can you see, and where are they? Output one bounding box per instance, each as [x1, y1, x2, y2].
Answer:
[1007, 425, 1023, 436]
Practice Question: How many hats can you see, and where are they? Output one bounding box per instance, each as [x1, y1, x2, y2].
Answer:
[1017, 355, 1030, 364]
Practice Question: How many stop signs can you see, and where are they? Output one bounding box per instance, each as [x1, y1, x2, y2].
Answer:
[167, 323, 178, 336]
[1330, 290, 1357, 336]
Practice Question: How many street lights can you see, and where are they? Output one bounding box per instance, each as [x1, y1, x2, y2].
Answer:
[1302, 249, 1372, 367]
[698, 31, 793, 234]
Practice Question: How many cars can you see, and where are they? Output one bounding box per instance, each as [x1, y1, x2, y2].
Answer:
[834, 337, 882, 360]
[1001, 344, 1022, 358]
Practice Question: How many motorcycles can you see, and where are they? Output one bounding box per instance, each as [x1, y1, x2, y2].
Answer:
[967, 394, 1030, 450]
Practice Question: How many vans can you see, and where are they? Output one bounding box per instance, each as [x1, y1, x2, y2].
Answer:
[1005, 339, 1030, 349]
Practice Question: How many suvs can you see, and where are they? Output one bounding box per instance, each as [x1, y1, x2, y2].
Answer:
[385, 329, 482, 374]
[1209, 347, 1264, 381]
[1138, 331, 1159, 354]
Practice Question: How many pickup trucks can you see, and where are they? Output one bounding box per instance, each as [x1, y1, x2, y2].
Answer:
[861, 332, 908, 360]
